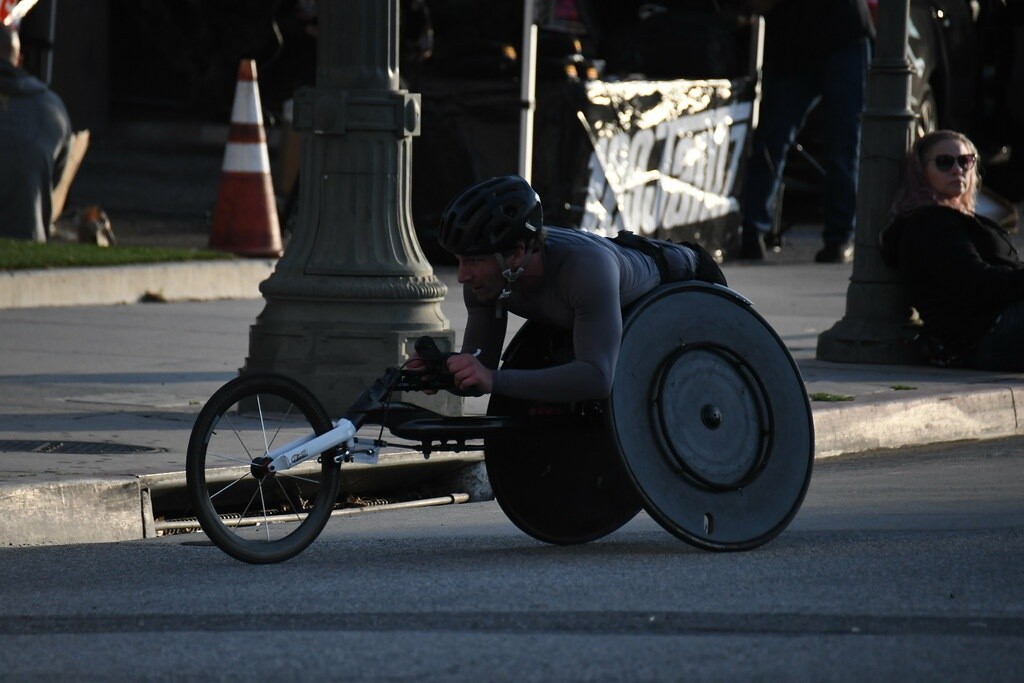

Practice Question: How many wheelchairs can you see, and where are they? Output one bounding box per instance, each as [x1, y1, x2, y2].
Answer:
[185, 279, 816, 566]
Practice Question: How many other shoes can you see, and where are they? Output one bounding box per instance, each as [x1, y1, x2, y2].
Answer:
[814, 233, 854, 264]
[712, 231, 774, 262]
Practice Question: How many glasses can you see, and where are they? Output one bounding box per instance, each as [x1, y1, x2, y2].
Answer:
[928, 151, 976, 171]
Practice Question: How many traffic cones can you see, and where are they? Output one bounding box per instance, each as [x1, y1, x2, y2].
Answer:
[207, 57, 286, 260]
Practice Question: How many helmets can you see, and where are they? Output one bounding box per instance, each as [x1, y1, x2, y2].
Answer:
[436, 174, 543, 255]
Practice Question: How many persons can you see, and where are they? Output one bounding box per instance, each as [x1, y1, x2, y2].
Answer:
[299, 0, 436, 62]
[711, 0, 876, 266]
[407, 169, 727, 403]
[882, 131, 1023, 371]
